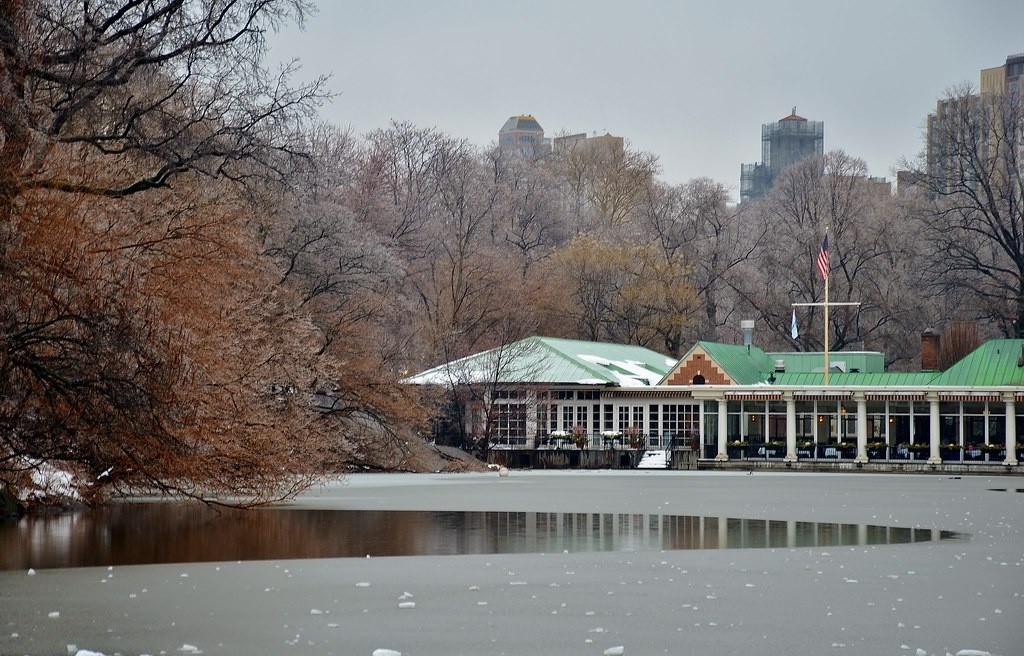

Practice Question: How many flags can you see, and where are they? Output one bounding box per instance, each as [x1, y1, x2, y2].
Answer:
[817, 229, 831, 281]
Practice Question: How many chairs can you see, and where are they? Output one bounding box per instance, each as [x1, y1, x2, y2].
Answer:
[545, 430, 643, 450]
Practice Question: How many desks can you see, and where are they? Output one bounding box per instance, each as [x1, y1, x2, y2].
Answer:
[966, 450, 981, 458]
[796, 447, 810, 457]
[826, 448, 838, 458]
[759, 446, 776, 458]
[602, 431, 622, 450]
[552, 431, 569, 449]
[898, 447, 920, 460]
